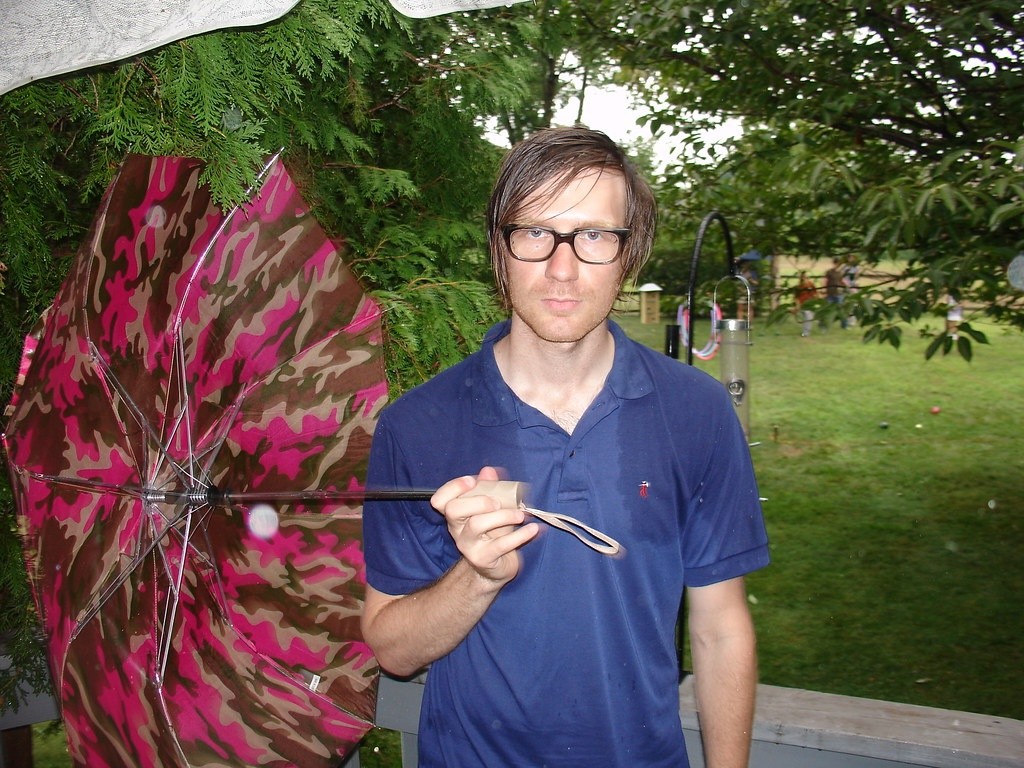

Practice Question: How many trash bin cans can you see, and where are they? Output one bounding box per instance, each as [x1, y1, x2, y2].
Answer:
[638, 282, 663, 324]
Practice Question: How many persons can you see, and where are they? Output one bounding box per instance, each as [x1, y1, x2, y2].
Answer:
[0, 138, 523, 768]
[731, 255, 962, 340]
[363, 126, 771, 768]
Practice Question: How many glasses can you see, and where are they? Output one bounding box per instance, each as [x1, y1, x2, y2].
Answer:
[500, 223, 635, 265]
